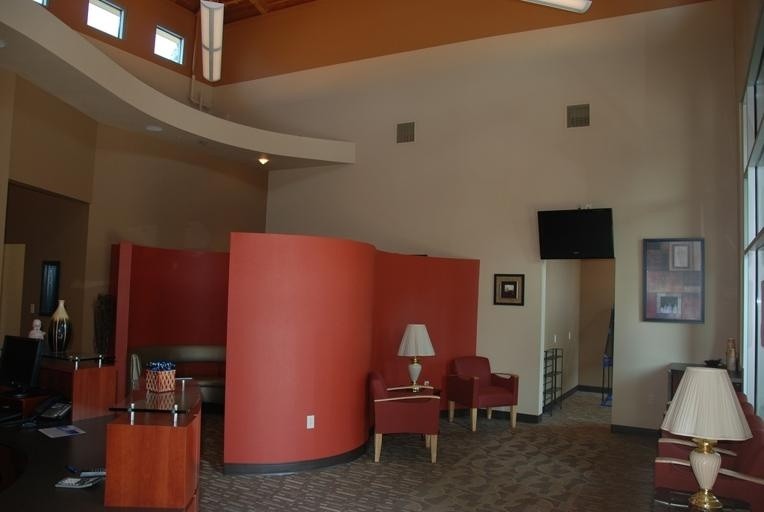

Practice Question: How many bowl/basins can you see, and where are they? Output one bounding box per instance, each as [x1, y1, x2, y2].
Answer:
[704, 358, 722, 366]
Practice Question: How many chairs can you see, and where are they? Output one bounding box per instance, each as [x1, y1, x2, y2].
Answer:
[655, 391, 764, 512]
[446, 356, 519, 432]
[368, 370, 440, 463]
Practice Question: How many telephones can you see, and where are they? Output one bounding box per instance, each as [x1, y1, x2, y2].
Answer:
[35, 394, 73, 421]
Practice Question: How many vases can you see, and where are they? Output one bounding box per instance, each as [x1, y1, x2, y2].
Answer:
[48, 300, 71, 353]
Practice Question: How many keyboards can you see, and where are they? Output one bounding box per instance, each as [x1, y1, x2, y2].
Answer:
[0, 408, 22, 422]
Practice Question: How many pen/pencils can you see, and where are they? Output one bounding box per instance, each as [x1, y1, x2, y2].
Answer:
[66, 464, 77, 473]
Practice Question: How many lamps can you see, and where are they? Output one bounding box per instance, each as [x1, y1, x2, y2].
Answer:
[660, 366, 753, 512]
[521, 0, 593, 14]
[200, 0, 225, 82]
[397, 325, 436, 392]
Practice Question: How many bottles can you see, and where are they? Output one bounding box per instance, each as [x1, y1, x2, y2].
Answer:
[726, 338, 737, 372]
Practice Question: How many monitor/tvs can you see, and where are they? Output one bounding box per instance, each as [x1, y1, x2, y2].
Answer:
[536, 207, 615, 260]
[0, 334, 44, 399]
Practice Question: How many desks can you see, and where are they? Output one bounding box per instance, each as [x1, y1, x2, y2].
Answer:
[668, 363, 743, 400]
[400, 385, 442, 441]
[0, 413, 184, 512]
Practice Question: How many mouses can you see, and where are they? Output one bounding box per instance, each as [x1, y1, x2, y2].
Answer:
[16, 422, 37, 429]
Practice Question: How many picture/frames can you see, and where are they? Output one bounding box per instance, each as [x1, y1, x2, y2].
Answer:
[39, 261, 60, 316]
[642, 238, 706, 324]
[494, 273, 525, 306]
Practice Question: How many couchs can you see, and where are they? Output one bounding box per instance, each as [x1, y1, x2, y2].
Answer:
[131, 345, 226, 405]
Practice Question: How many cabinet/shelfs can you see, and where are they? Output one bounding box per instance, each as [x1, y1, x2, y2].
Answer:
[103, 383, 201, 507]
[544, 348, 563, 416]
[39, 365, 119, 421]
